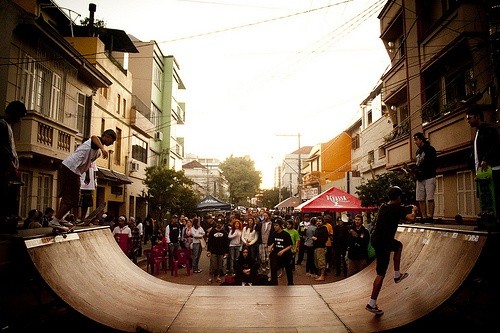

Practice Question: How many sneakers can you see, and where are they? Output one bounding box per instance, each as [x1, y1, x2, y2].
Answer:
[365, 304, 383, 314]
[394, 272, 409, 283]
[75, 219, 85, 225]
[48, 218, 73, 230]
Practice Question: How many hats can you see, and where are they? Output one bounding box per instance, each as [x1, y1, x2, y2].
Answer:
[413, 131, 429, 139]
[216, 218, 224, 223]
[172, 214, 179, 218]
[119, 215, 125, 221]
[273, 218, 284, 229]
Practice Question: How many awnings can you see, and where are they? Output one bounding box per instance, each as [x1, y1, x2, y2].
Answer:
[97, 167, 133, 185]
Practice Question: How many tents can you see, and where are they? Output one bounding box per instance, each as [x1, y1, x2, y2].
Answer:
[294, 187, 379, 213]
[191, 195, 234, 212]
[274, 197, 305, 209]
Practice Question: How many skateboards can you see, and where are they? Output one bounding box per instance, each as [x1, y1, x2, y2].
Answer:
[80, 201, 106, 225]
[474, 165, 498, 223]
[49, 220, 76, 234]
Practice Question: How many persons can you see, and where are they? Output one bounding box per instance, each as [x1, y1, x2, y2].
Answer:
[0, 99, 28, 234]
[407, 133, 437, 224]
[48, 129, 116, 231]
[78, 137, 98, 225]
[112, 215, 132, 260]
[364, 186, 419, 314]
[127, 207, 382, 287]
[466, 107, 500, 232]
[99, 210, 115, 232]
[23, 207, 55, 228]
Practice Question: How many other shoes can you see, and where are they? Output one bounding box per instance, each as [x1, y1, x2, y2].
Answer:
[193, 268, 202, 273]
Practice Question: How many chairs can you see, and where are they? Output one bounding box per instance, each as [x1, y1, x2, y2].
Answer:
[143, 246, 191, 277]
